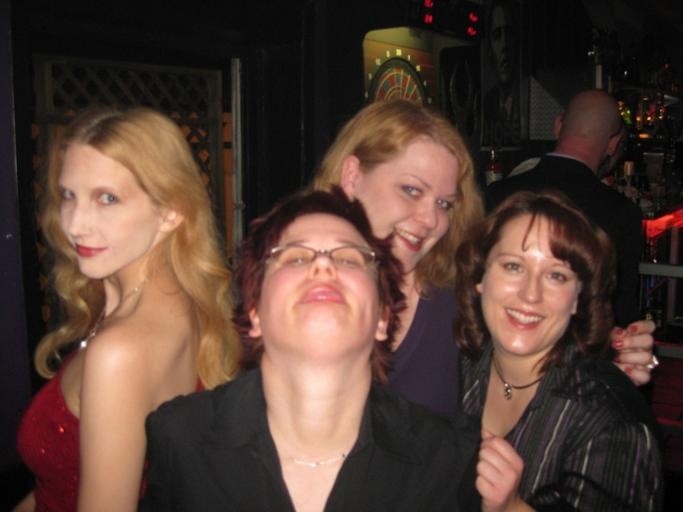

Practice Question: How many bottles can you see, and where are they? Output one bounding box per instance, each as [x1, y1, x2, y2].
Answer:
[486, 149, 503, 193]
[617, 161, 641, 206]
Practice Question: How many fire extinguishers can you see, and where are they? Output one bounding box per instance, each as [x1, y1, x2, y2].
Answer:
[483, 147, 504, 186]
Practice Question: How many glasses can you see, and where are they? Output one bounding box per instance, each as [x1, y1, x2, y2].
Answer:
[268, 244, 376, 270]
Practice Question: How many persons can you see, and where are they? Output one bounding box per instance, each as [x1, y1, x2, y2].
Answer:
[459, 187, 662, 512]
[12, 106, 242, 509]
[483, 88, 644, 327]
[141, 182, 471, 512]
[313, 98, 661, 437]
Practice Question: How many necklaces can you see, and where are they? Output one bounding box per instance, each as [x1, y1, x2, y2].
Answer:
[490, 358, 547, 398]
[78, 271, 156, 350]
[274, 455, 346, 466]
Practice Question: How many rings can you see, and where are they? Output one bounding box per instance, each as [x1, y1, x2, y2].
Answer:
[645, 357, 660, 369]
